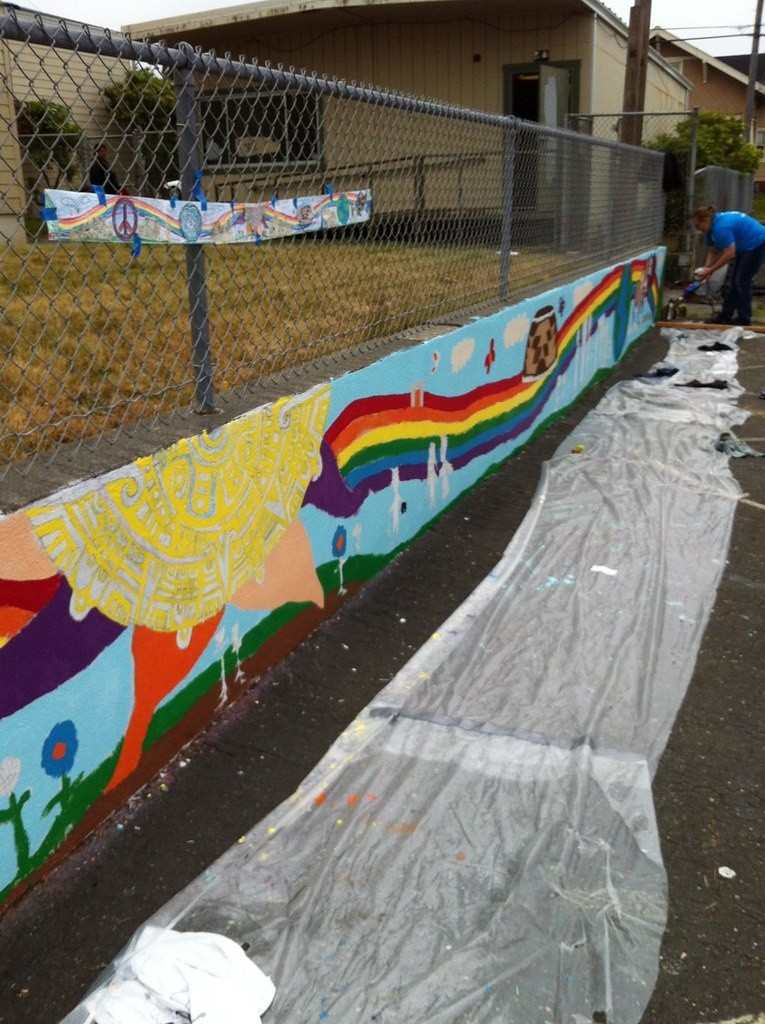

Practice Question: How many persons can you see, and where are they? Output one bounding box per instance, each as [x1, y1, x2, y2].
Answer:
[691, 205, 765, 326]
[90, 143, 121, 195]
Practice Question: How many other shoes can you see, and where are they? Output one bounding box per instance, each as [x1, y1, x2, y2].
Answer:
[727, 317, 751, 326]
[704, 317, 725, 324]
